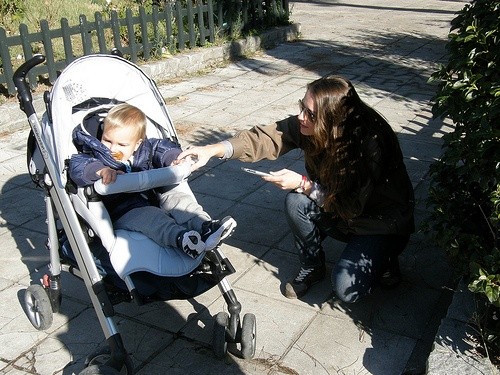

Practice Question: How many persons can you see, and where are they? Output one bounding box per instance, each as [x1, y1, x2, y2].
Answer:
[176, 74, 416, 304]
[67, 104, 238, 259]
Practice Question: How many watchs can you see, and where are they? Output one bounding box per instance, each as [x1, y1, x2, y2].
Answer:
[295, 175, 307, 194]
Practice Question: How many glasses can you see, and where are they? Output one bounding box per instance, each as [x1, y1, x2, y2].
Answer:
[298, 99, 316, 123]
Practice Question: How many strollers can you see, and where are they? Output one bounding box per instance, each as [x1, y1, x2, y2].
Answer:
[13, 47, 256, 374]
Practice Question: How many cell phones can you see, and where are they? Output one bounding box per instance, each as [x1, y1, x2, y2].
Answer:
[241, 167, 274, 177]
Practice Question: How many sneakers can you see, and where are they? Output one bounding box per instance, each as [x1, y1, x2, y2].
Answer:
[178, 229, 206, 260]
[380, 255, 400, 289]
[200, 215, 238, 253]
[284, 266, 321, 300]
